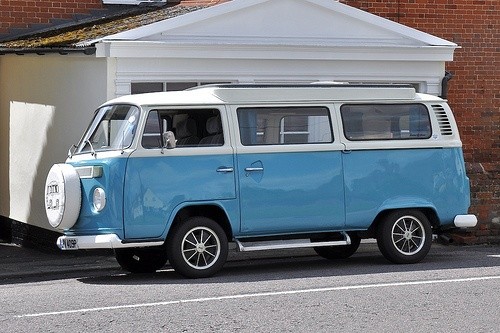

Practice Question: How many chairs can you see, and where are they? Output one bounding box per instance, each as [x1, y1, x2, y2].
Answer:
[175, 117, 224, 147]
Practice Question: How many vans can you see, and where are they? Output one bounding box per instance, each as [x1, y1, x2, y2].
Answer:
[45, 83, 477, 277]
